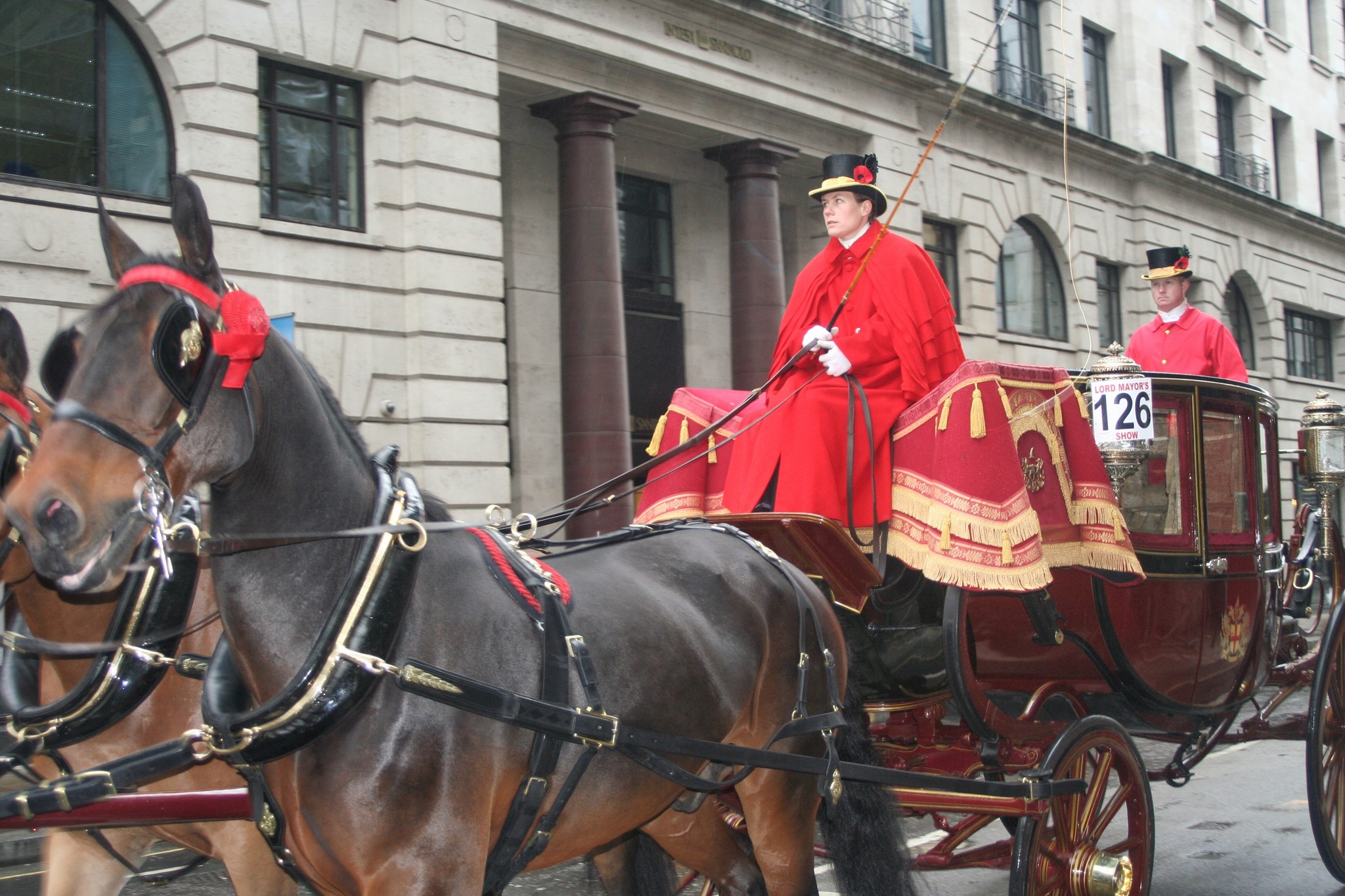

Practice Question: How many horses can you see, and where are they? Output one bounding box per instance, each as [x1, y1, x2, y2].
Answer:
[0, 173, 932, 896]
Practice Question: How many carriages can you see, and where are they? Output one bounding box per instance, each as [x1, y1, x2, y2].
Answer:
[0, 171, 1345, 896]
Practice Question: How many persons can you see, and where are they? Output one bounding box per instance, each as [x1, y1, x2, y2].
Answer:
[1125, 244, 1249, 384]
[721, 153, 967, 525]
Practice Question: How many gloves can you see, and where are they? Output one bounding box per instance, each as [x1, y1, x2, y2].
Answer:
[817, 339, 851, 377]
[802, 325, 839, 352]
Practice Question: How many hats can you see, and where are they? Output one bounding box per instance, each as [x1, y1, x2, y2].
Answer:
[1141, 244, 1193, 280]
[808, 153, 889, 218]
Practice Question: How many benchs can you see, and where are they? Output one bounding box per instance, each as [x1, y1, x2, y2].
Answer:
[630, 360, 1146, 593]
